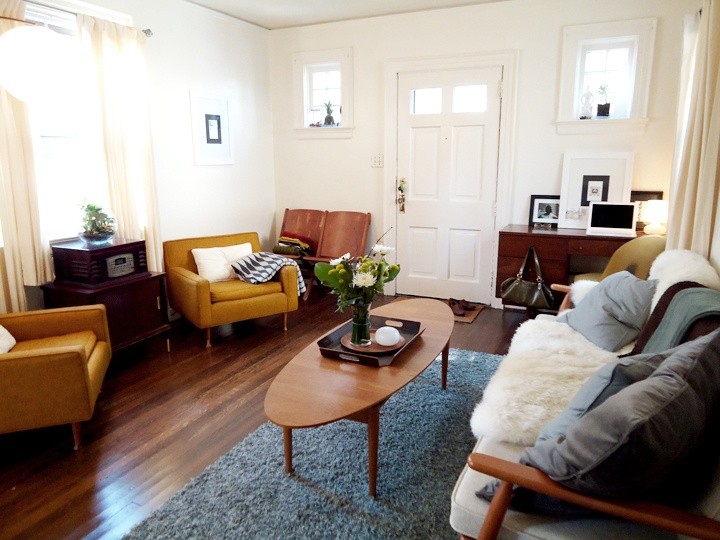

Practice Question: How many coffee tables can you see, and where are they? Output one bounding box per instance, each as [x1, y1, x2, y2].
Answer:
[264, 297, 455, 497]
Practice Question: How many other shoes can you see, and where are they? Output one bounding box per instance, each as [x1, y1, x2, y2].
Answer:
[457, 299, 475, 310]
[449, 298, 465, 316]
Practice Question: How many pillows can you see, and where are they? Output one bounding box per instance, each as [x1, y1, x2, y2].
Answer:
[0, 325, 16, 353]
[474, 271, 719, 520]
[191, 243, 253, 282]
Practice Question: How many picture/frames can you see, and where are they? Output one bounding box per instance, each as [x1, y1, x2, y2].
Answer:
[205, 114, 221, 144]
[529, 195, 560, 228]
[630, 191, 663, 230]
[558, 151, 634, 230]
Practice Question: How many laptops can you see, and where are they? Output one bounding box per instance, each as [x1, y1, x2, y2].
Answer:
[586, 200, 640, 240]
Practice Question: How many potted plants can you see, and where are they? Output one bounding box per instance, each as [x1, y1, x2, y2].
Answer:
[78, 204, 117, 244]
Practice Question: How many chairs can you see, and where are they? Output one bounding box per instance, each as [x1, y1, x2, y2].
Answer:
[276, 208, 371, 301]
[163, 232, 298, 346]
[574, 235, 666, 282]
[0, 304, 112, 450]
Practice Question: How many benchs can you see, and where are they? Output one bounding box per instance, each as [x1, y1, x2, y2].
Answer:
[450, 250, 720, 540]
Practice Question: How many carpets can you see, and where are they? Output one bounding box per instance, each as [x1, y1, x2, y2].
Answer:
[121, 347, 505, 540]
[392, 295, 485, 323]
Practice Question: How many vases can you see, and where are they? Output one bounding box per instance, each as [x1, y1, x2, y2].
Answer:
[350, 302, 373, 347]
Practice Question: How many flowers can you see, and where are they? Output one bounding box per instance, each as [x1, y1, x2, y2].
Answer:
[314, 227, 401, 345]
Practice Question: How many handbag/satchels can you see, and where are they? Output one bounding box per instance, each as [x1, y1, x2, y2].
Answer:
[500, 247, 554, 310]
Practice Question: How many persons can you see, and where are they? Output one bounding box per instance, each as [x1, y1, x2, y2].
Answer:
[537, 205, 559, 219]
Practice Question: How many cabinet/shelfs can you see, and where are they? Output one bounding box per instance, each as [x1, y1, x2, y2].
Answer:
[40, 235, 171, 353]
[496, 224, 646, 319]
